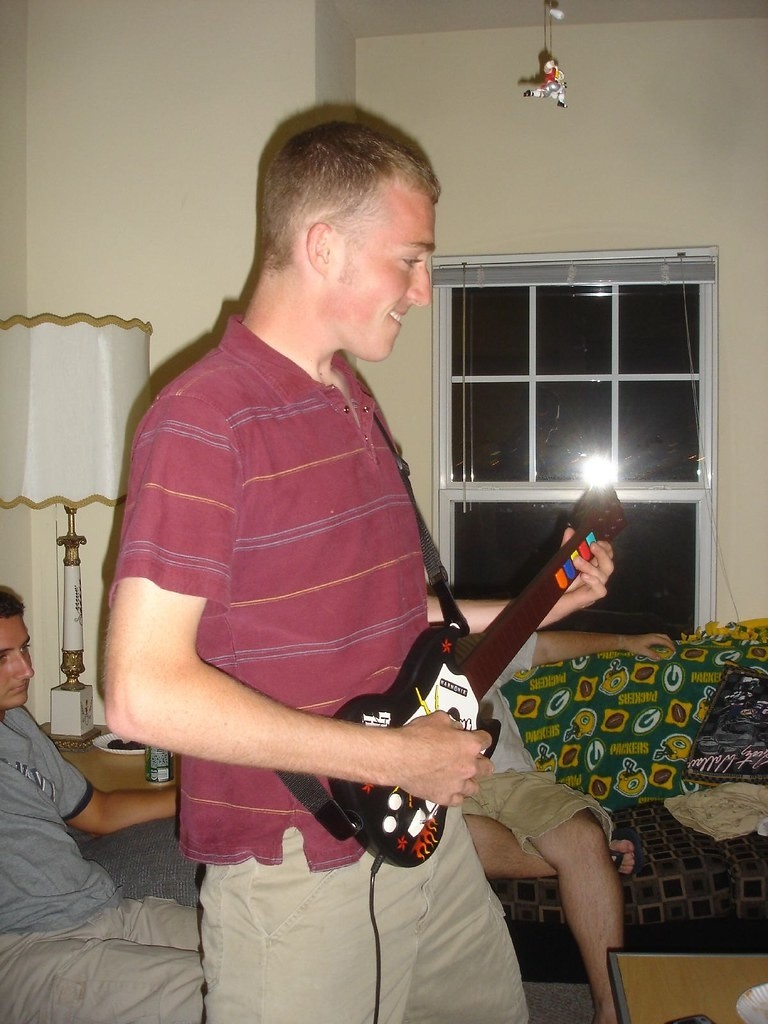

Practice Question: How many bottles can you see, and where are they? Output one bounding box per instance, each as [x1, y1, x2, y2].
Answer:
[144, 744, 175, 786]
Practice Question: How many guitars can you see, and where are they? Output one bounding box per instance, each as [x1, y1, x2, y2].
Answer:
[321, 479, 630, 872]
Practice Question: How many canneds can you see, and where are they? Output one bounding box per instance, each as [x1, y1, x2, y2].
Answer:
[144, 744, 174, 785]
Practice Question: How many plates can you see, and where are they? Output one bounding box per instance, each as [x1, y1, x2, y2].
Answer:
[736, 982, 768, 1024]
[92, 733, 145, 754]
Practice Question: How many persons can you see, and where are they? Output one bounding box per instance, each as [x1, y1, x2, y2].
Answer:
[106, 121, 613, 1024]
[0, 591, 206, 1024]
[461, 631, 674, 1023]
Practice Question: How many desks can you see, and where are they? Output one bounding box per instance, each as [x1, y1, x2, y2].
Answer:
[32, 721, 180, 794]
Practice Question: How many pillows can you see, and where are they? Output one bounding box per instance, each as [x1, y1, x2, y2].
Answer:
[485, 617, 768, 813]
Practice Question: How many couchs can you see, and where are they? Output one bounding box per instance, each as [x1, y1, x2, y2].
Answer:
[492, 799, 768, 954]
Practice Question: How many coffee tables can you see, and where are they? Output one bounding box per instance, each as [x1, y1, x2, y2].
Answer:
[606, 951, 768, 1024]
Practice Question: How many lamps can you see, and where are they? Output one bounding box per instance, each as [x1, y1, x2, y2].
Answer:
[0, 311, 155, 754]
